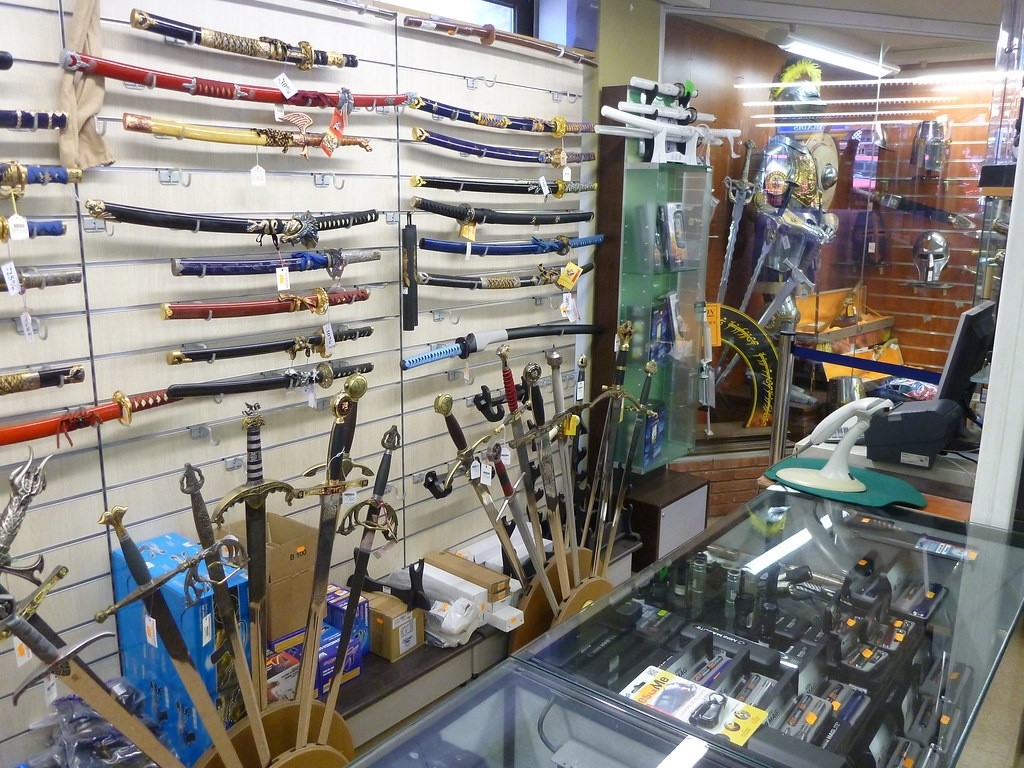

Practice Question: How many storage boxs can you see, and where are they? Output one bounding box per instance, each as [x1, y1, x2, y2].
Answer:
[110, 499, 554, 768]
